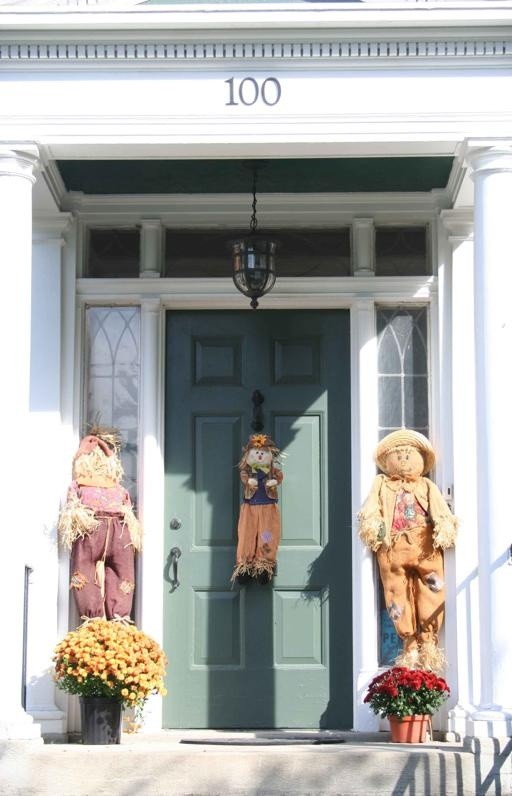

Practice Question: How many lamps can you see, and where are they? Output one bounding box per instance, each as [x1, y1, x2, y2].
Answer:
[225, 156, 287, 311]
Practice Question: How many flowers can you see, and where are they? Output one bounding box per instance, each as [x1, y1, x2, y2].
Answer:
[50, 616, 168, 734]
[363, 666, 451, 722]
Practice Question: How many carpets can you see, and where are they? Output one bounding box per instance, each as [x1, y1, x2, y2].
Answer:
[181, 737, 348, 744]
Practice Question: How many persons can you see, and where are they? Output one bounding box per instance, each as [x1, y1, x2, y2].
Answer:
[357, 429, 458, 671]
[59, 410, 144, 627]
[230, 432, 290, 586]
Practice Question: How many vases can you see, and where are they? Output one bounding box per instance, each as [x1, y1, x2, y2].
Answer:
[389, 714, 430, 743]
[78, 695, 121, 745]
